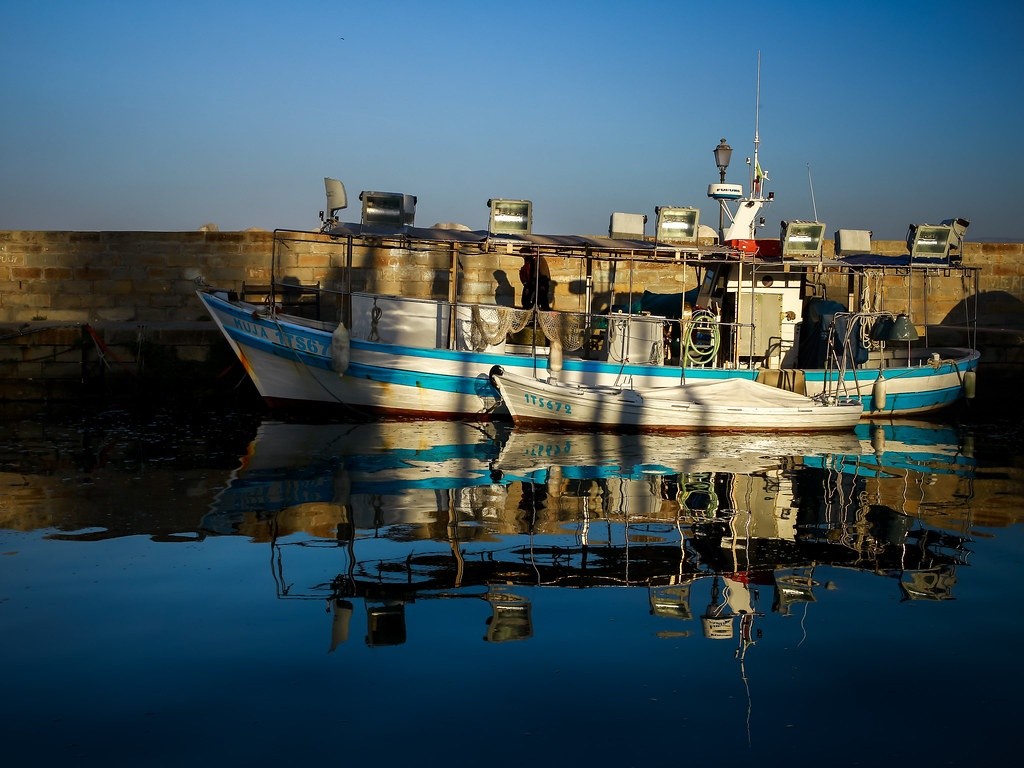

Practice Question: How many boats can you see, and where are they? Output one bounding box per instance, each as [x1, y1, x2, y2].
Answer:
[486, 364, 865, 436]
[494, 428, 865, 514]
[203, 408, 971, 635]
[186, 167, 989, 421]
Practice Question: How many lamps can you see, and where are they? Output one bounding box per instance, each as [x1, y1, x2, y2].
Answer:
[833, 229, 873, 258]
[780, 220, 826, 262]
[324, 178, 347, 222]
[359, 191, 404, 233]
[608, 212, 647, 241]
[939, 218, 970, 258]
[906, 223, 953, 267]
[869, 315, 895, 341]
[649, 584, 693, 622]
[485, 198, 533, 243]
[404, 195, 417, 228]
[328, 598, 353, 653]
[888, 313, 919, 341]
[364, 599, 406, 647]
[483, 598, 533, 642]
[655, 206, 700, 250]
[772, 568, 817, 608]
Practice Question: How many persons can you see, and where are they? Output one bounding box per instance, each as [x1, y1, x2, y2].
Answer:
[519, 248, 535, 307]
[531, 249, 550, 310]
